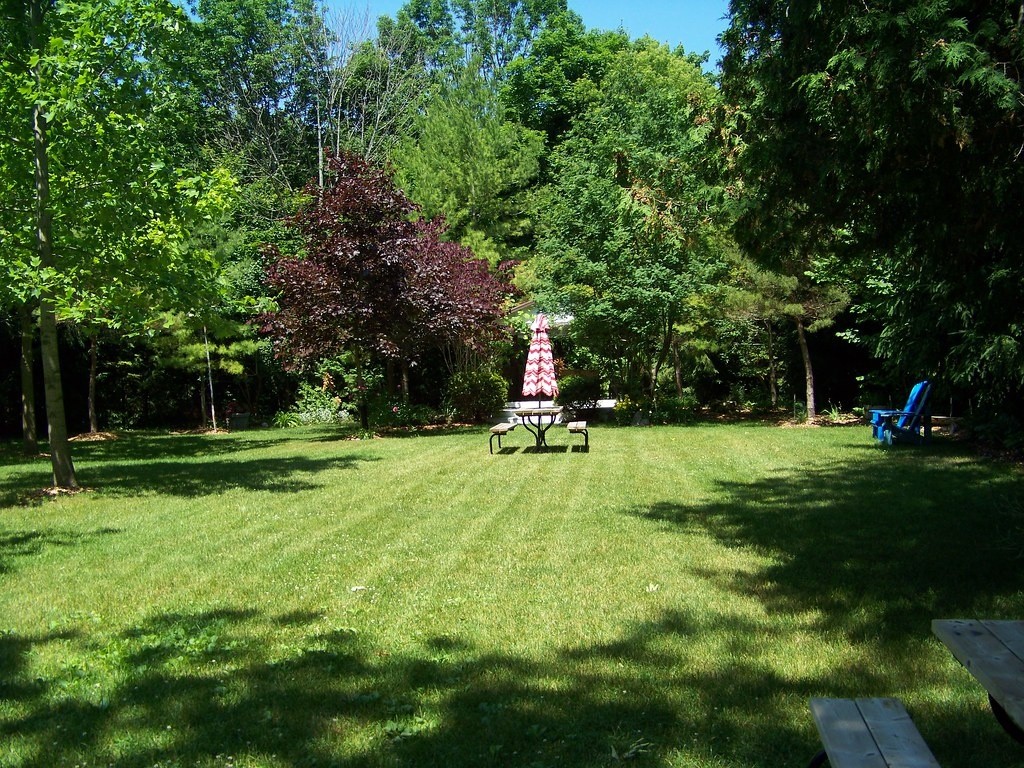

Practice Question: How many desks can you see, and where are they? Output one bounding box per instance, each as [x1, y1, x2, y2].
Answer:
[931, 619, 1024, 748]
[514, 406, 564, 452]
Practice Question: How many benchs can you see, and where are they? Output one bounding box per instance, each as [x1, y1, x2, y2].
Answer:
[869, 381, 934, 445]
[567, 421, 590, 452]
[808, 697, 941, 768]
[489, 422, 518, 454]
